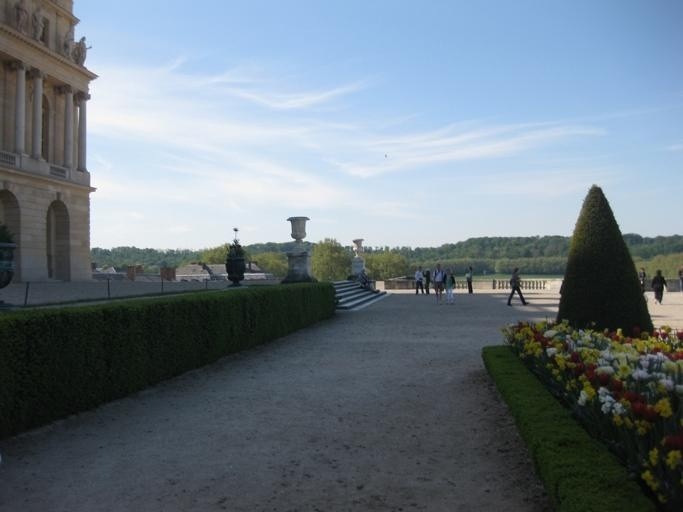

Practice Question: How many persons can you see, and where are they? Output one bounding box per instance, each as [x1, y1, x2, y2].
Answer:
[651, 269, 668, 304]
[639, 267, 645, 293]
[465, 266, 474, 295]
[415, 267, 425, 295]
[423, 270, 431, 294]
[507, 267, 529, 306]
[443, 269, 455, 306]
[433, 263, 445, 305]
[359, 268, 381, 293]
